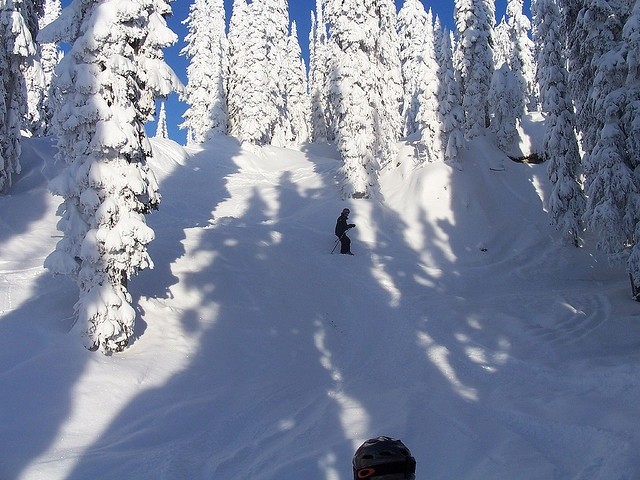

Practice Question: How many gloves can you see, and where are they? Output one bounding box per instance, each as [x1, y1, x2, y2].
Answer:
[349, 224, 355, 227]
[348, 223, 352, 228]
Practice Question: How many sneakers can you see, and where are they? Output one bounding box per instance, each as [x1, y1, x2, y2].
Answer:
[346, 251, 354, 256]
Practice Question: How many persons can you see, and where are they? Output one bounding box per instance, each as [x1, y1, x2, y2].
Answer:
[334, 207, 356, 256]
